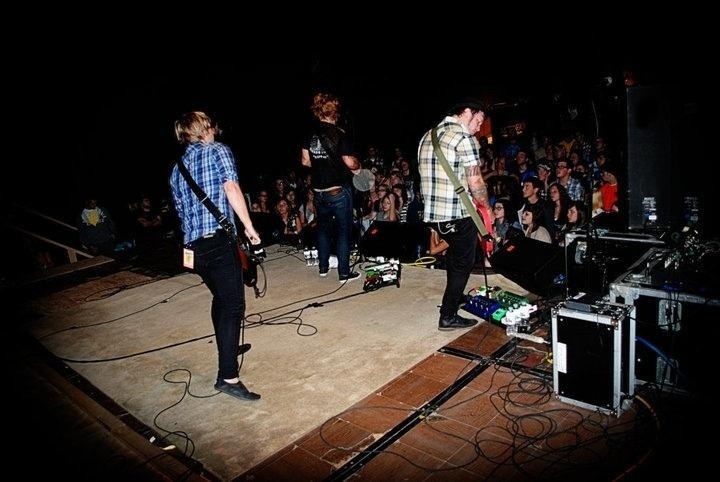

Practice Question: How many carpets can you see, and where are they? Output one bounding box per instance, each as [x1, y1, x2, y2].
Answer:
[18, 243, 529, 482]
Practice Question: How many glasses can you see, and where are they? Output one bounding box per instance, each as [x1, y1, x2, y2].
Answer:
[555, 164, 567, 170]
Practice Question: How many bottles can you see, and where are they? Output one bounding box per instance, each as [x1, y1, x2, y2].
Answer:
[303, 245, 319, 268]
[506, 300, 529, 337]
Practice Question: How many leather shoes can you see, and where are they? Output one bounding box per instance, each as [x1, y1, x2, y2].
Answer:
[237, 343, 251, 357]
[214, 378, 261, 402]
[439, 313, 479, 330]
[460, 293, 473, 307]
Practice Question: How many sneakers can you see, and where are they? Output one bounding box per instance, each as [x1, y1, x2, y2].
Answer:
[317, 268, 331, 277]
[338, 271, 361, 285]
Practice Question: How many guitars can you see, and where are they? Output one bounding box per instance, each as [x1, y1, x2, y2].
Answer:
[234, 194, 257, 287]
[470, 136, 495, 251]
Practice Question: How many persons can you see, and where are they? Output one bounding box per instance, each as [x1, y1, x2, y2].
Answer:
[168, 110, 262, 401]
[301, 83, 362, 282]
[416, 102, 494, 330]
[69, 122, 619, 259]
